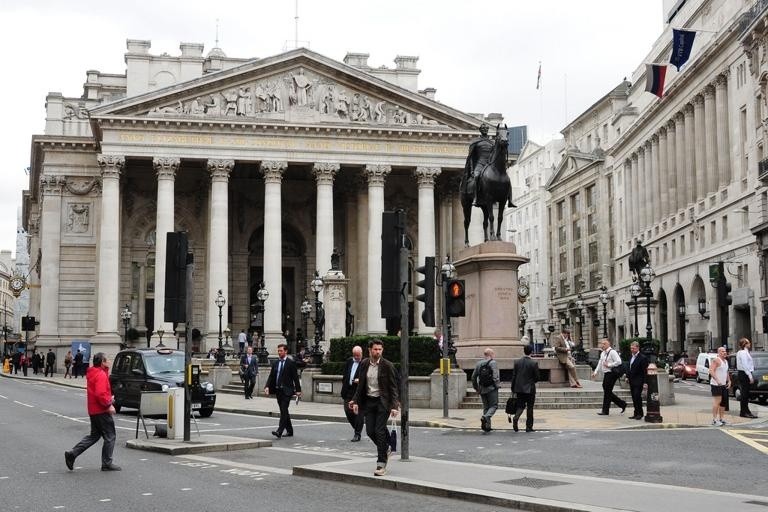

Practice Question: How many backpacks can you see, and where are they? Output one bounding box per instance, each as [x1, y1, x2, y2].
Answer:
[479, 359, 494, 388]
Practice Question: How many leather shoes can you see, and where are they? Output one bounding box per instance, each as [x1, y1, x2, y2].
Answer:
[597, 412, 609, 415]
[629, 415, 643, 420]
[621, 403, 627, 413]
[480, 416, 494, 432]
[740, 413, 757, 418]
[272, 431, 281, 438]
[351, 436, 360, 442]
[282, 433, 293, 437]
[513, 419, 518, 432]
[526, 427, 536, 432]
[571, 382, 583, 388]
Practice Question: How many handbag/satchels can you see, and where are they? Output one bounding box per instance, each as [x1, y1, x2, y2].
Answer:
[239, 366, 246, 381]
[391, 429, 396, 452]
[506, 398, 518, 414]
[611, 362, 625, 378]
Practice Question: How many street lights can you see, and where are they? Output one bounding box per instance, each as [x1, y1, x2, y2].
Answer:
[598, 285, 610, 338]
[575, 298, 584, 363]
[214, 288, 226, 365]
[628, 283, 642, 338]
[639, 266, 657, 339]
[120, 307, 132, 345]
[156, 325, 166, 346]
[255, 282, 270, 368]
[441, 256, 457, 420]
[298, 270, 325, 367]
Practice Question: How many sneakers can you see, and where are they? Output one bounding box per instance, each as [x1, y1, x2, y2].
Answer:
[721, 419, 732, 425]
[712, 419, 723, 426]
[65, 452, 75, 470]
[102, 465, 121, 471]
[386, 445, 392, 456]
[374, 466, 386, 476]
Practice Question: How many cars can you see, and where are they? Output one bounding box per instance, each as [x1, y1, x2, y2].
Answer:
[673, 357, 697, 380]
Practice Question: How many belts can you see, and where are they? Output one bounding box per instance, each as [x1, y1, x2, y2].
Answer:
[276, 386, 283, 389]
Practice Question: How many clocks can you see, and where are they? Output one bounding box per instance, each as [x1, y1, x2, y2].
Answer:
[8, 268, 26, 298]
[517, 276, 529, 304]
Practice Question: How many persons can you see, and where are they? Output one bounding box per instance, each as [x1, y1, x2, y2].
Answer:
[512, 344, 541, 433]
[161, 66, 406, 124]
[460, 122, 518, 208]
[313, 301, 325, 340]
[4, 345, 56, 377]
[554, 329, 583, 389]
[62, 351, 124, 472]
[735, 338, 759, 419]
[433, 328, 444, 357]
[207, 348, 220, 359]
[471, 348, 501, 432]
[74, 349, 84, 379]
[293, 347, 310, 378]
[340, 345, 367, 442]
[628, 239, 650, 275]
[237, 328, 248, 355]
[591, 338, 628, 415]
[239, 346, 258, 399]
[264, 344, 304, 437]
[346, 301, 354, 337]
[625, 341, 648, 420]
[708, 347, 732, 427]
[64, 349, 73, 379]
[248, 332, 262, 348]
[346, 339, 400, 477]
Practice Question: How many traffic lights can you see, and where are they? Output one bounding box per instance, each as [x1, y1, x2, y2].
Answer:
[718, 281, 732, 312]
[416, 257, 434, 327]
[447, 280, 464, 316]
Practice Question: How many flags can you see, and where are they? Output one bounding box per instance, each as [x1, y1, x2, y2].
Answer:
[644, 63, 668, 98]
[669, 29, 696, 72]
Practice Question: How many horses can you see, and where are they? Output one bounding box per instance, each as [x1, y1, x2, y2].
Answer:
[459, 124, 511, 249]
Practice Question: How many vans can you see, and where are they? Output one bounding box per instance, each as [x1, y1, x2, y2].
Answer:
[584, 348, 603, 367]
[108, 350, 215, 418]
[696, 353, 768, 402]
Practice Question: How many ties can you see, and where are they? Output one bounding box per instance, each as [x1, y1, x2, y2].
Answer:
[278, 360, 284, 386]
